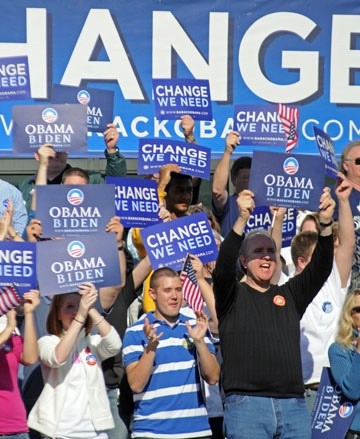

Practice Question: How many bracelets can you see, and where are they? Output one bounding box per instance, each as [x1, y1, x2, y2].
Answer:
[92, 316, 104, 325]
[319, 220, 333, 226]
[117, 241, 124, 250]
[71, 318, 85, 326]
[10, 231, 19, 240]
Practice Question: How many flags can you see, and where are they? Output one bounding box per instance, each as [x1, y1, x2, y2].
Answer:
[179, 255, 206, 316]
[277, 104, 298, 154]
[0, 282, 24, 317]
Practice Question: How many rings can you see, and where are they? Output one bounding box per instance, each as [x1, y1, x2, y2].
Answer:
[148, 336, 153, 340]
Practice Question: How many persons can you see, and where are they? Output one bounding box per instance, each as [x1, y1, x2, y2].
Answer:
[0, 115, 360, 439]
[0, 289, 41, 439]
[211, 186, 334, 439]
[27, 283, 123, 439]
[121, 267, 222, 439]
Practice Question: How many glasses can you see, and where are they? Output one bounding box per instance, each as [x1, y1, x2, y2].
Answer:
[345, 157, 360, 165]
[352, 306, 360, 313]
